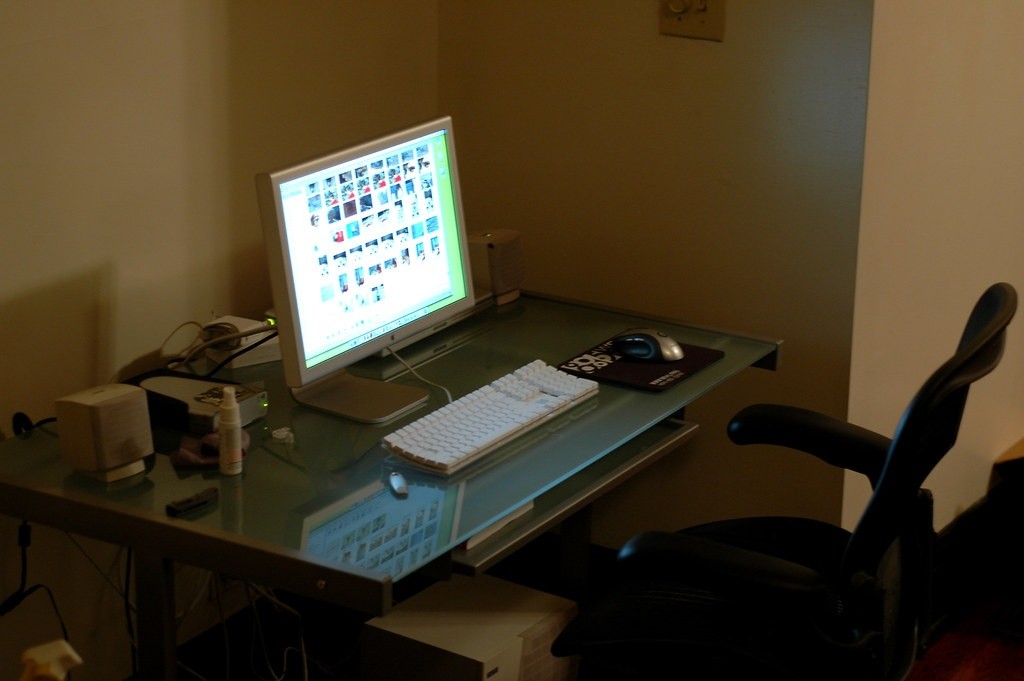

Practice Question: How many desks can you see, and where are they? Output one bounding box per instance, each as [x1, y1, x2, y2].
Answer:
[0, 289, 784, 681]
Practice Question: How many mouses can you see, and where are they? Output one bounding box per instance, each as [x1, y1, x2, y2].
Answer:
[612, 327, 685, 365]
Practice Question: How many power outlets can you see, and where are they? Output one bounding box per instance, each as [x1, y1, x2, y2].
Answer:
[209, 569, 235, 601]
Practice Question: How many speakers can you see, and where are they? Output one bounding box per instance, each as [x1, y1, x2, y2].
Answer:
[466, 228, 524, 306]
[56, 383, 153, 483]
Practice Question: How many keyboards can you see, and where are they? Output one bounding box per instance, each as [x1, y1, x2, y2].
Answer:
[381, 358, 600, 476]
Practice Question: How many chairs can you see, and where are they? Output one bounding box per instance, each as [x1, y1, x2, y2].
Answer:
[554, 281, 1019, 681]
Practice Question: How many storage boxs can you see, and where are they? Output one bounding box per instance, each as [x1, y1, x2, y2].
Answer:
[360, 572, 580, 681]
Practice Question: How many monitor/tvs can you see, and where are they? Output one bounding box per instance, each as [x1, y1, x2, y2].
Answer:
[254, 113, 478, 423]
